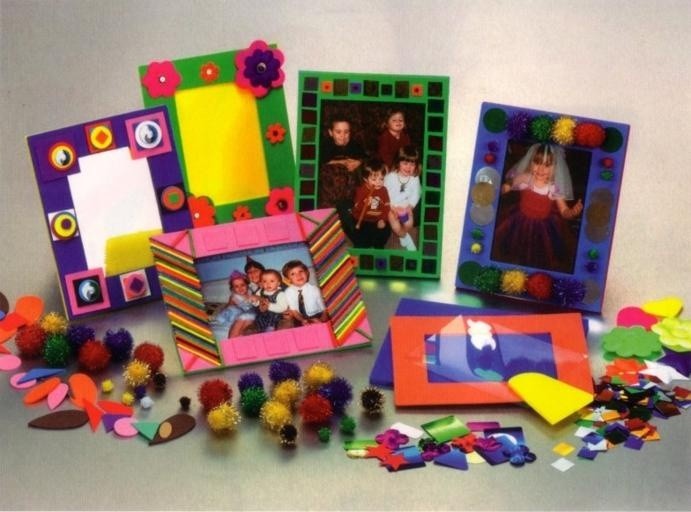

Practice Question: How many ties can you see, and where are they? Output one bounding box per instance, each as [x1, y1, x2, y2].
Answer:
[297, 291, 309, 319]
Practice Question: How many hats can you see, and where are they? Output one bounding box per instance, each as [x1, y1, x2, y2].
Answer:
[229, 269, 249, 283]
[244, 256, 265, 272]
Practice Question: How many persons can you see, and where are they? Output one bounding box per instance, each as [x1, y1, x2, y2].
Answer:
[319, 108, 422, 252]
[215, 260, 325, 339]
[490, 143, 584, 275]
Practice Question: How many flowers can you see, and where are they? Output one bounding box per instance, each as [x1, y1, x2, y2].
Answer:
[232, 37, 284, 98]
[184, 190, 217, 229]
[231, 205, 252, 220]
[141, 60, 179, 99]
[265, 122, 287, 145]
[263, 186, 295, 217]
[199, 61, 218, 83]
